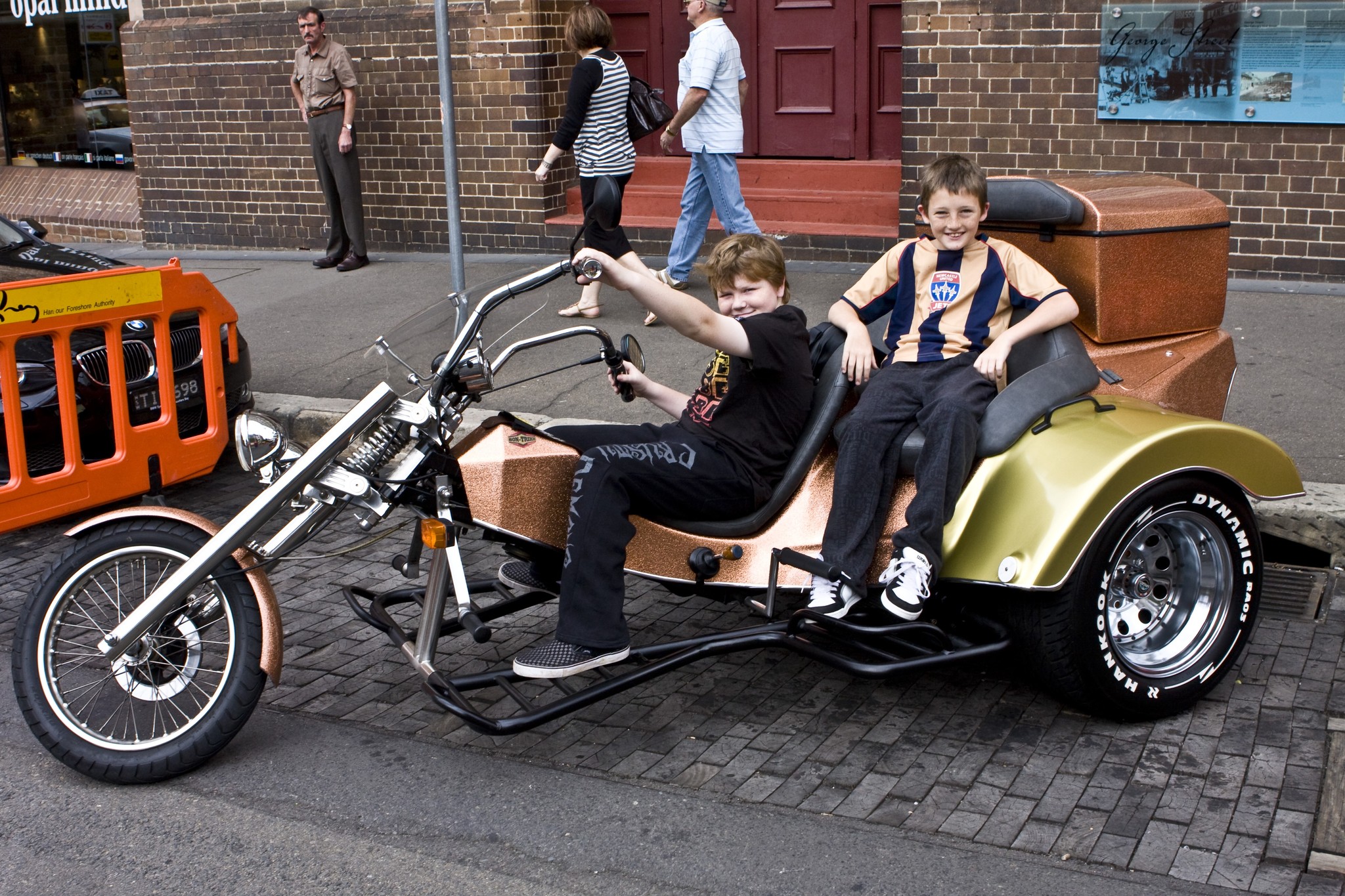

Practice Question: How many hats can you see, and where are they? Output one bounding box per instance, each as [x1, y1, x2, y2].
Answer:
[706, 0, 727, 7]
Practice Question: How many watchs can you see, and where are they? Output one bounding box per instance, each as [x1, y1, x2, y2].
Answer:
[666, 125, 678, 137]
[649, 0, 763, 290]
[343, 124, 352, 129]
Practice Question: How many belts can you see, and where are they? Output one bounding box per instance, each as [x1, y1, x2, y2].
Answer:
[307, 105, 344, 118]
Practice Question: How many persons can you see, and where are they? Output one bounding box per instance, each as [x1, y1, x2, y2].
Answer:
[535, 4, 659, 326]
[1106, 56, 1233, 98]
[804, 152, 1080, 628]
[290, 6, 370, 271]
[1264, 81, 1292, 102]
[497, 232, 817, 678]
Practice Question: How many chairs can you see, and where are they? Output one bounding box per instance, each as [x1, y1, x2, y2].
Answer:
[831, 179, 1099, 477]
[644, 324, 848, 541]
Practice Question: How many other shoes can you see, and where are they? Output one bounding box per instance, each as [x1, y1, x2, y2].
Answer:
[497, 560, 562, 598]
[513, 638, 630, 678]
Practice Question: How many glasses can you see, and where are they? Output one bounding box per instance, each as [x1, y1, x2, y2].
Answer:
[684, 0, 699, 6]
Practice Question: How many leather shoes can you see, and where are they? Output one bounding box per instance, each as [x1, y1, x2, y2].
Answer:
[313, 256, 344, 268]
[336, 250, 369, 270]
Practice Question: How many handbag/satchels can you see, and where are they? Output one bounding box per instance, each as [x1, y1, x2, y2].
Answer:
[626, 75, 674, 143]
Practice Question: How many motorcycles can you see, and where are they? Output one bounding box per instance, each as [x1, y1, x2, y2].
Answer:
[9, 176, 1338, 788]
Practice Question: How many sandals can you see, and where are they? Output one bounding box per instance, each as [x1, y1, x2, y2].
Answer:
[558, 302, 604, 319]
[644, 310, 657, 326]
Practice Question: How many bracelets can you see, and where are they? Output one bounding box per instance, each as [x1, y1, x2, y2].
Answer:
[542, 158, 552, 168]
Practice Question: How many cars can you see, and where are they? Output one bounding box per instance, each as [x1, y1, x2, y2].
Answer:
[0, 215, 255, 490]
[65, 87, 135, 164]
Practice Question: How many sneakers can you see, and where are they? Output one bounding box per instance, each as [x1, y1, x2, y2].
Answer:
[649, 268, 688, 289]
[878, 546, 932, 621]
[800, 553, 863, 624]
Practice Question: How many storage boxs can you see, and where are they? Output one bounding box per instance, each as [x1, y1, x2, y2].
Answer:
[915, 168, 1231, 346]
